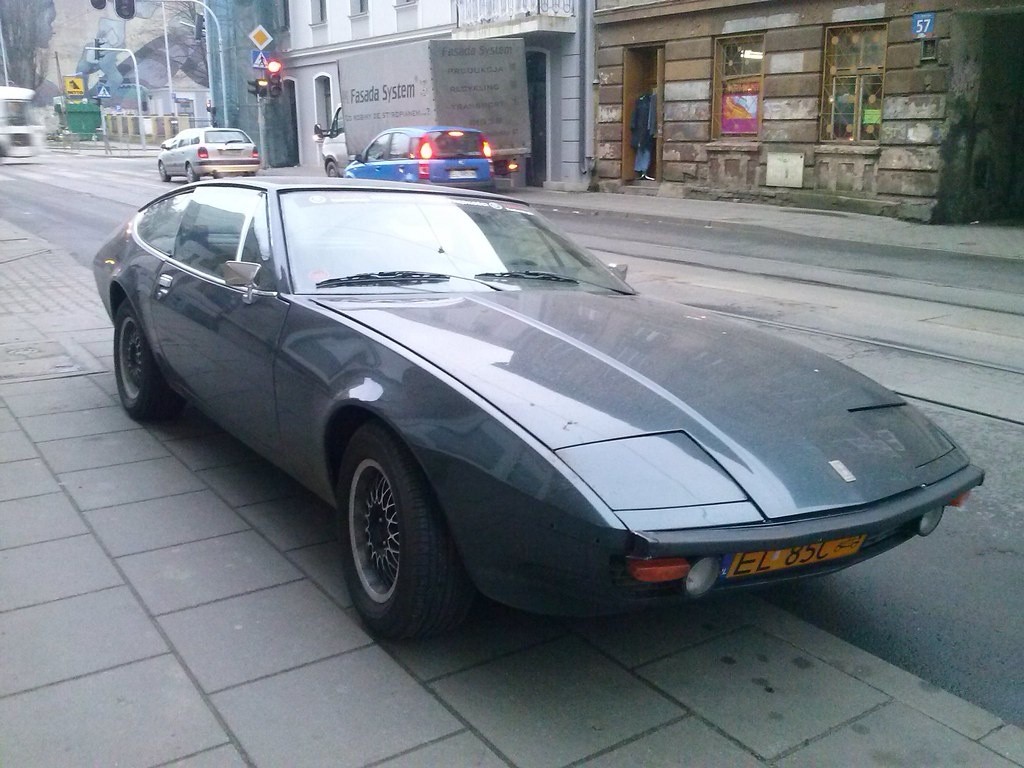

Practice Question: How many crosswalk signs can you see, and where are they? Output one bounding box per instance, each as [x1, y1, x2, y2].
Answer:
[251, 49, 271, 69]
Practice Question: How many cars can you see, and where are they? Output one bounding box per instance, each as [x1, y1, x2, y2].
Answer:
[342, 125, 496, 192]
[157, 127, 262, 184]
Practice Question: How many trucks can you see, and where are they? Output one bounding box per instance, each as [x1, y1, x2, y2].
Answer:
[314, 35, 532, 178]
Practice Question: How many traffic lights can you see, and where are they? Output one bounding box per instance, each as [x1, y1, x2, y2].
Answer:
[94, 39, 105, 60]
[268, 60, 283, 99]
[246, 78, 269, 98]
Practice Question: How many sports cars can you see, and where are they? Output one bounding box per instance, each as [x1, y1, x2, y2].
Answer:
[92, 176, 987, 641]
